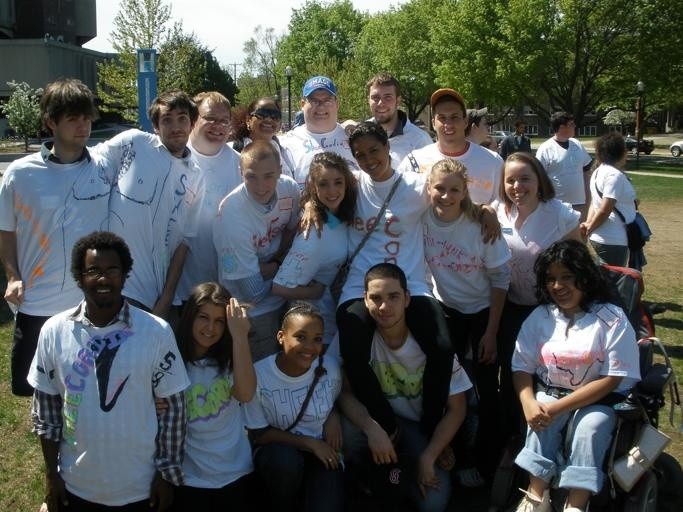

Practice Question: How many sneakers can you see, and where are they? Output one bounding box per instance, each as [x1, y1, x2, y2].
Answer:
[514, 484, 551, 512]
[456, 467, 495, 487]
[561, 496, 591, 512]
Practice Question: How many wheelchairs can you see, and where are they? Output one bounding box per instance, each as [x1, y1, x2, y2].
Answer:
[491, 266, 672, 511]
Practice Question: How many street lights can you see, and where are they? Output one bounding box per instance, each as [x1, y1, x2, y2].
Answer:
[283, 65, 295, 132]
[634, 81, 646, 171]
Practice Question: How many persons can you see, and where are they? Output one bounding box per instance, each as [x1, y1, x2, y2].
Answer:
[94, 92, 207, 318]
[422, 158, 513, 397]
[152, 281, 258, 512]
[225, 96, 299, 183]
[464, 107, 491, 145]
[0, 77, 119, 398]
[397, 87, 505, 206]
[489, 150, 583, 441]
[364, 73, 432, 172]
[579, 130, 641, 268]
[175, 89, 245, 318]
[213, 140, 301, 363]
[323, 261, 476, 511]
[480, 136, 500, 153]
[276, 75, 361, 193]
[297, 121, 502, 471]
[499, 120, 532, 160]
[269, 151, 359, 356]
[510, 239, 644, 511]
[25, 230, 190, 512]
[535, 111, 595, 224]
[240, 300, 348, 511]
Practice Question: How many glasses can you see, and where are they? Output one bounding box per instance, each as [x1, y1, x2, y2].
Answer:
[305, 99, 336, 108]
[80, 267, 122, 280]
[197, 114, 231, 127]
[248, 107, 281, 121]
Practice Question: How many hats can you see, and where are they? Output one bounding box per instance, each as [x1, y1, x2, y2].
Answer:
[430, 88, 465, 112]
[302, 76, 337, 98]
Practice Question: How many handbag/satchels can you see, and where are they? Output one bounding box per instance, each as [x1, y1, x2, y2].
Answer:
[329, 263, 350, 304]
[624, 212, 650, 252]
[608, 421, 672, 493]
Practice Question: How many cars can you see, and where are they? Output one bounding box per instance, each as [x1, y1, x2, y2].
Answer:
[623, 135, 654, 155]
[490, 131, 513, 145]
[671, 140, 682, 157]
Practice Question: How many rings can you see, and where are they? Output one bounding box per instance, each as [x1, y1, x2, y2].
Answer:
[537, 418, 543, 425]
[329, 458, 332, 463]
